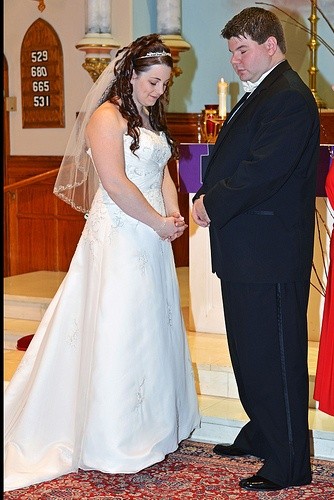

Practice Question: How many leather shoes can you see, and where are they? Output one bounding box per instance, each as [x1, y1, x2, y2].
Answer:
[240, 474, 287, 492]
[215, 443, 257, 457]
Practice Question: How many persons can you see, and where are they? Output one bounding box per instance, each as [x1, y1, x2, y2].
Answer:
[192, 7, 320, 491]
[4, 34, 200, 493]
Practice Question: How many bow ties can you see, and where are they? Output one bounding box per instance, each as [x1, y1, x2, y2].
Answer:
[242, 81, 256, 93]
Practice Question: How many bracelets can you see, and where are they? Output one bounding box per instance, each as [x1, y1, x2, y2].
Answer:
[154, 217, 167, 233]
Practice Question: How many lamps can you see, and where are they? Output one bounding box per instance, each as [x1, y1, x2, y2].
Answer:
[76, 0, 192, 86]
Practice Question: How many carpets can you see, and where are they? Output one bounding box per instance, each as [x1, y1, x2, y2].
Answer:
[3, 439, 334, 500]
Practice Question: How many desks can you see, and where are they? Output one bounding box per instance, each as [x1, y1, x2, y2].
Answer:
[179, 143, 334, 342]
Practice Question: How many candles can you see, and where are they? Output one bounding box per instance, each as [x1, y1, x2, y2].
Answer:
[217, 77, 229, 117]
